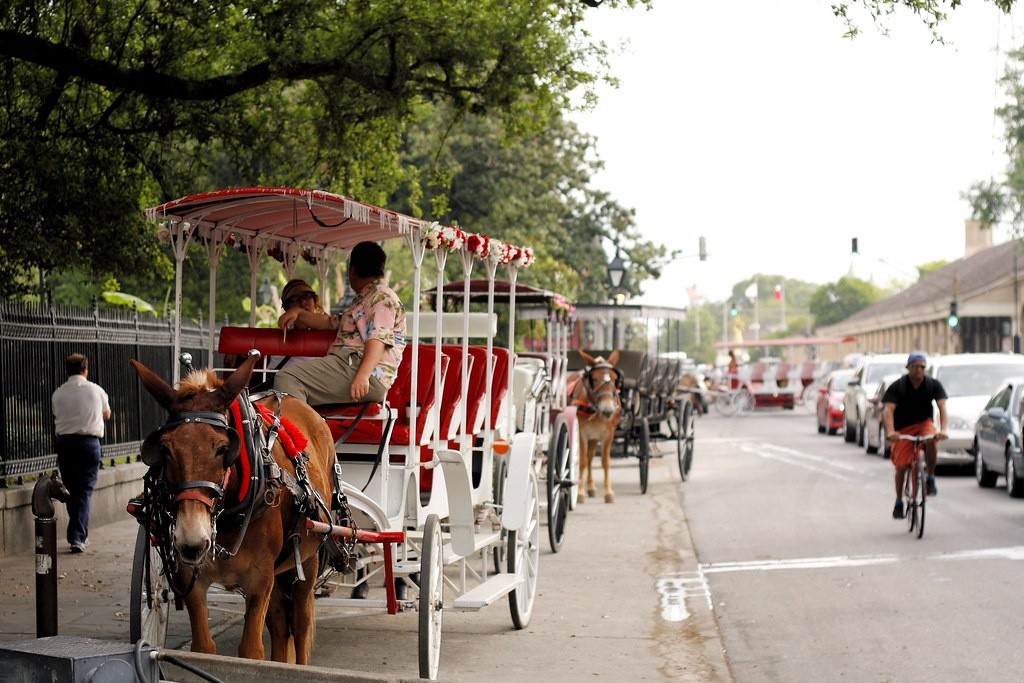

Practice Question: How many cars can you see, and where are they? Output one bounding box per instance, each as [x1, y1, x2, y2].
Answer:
[816, 352, 1024, 497]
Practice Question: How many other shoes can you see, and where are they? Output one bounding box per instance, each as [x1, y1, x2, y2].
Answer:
[70, 535, 89, 552]
[892, 500, 904, 518]
[925, 479, 937, 497]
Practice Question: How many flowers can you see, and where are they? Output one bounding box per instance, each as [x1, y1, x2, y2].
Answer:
[568, 305, 576, 313]
[492, 239, 512, 263]
[425, 222, 442, 250]
[552, 294, 565, 307]
[469, 235, 490, 259]
[514, 245, 533, 267]
[443, 225, 465, 252]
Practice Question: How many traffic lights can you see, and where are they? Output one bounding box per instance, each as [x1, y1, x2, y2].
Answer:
[948, 301, 959, 331]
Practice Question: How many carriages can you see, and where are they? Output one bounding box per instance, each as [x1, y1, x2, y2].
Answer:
[565, 302, 696, 493]
[125, 186, 580, 683]
[677, 337, 857, 417]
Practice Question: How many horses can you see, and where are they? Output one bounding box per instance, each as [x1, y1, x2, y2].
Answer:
[677, 374, 713, 415]
[130, 355, 336, 665]
[561, 349, 625, 503]
[32, 470, 70, 519]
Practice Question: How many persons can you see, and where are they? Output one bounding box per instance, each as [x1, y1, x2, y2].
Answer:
[52, 354, 111, 553]
[710, 361, 722, 389]
[881, 351, 949, 518]
[248, 282, 330, 393]
[728, 350, 739, 389]
[274, 239, 407, 407]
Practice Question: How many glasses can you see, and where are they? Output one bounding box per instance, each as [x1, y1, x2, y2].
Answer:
[911, 365, 926, 369]
[288, 293, 314, 305]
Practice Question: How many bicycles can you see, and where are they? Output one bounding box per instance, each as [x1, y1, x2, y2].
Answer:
[886, 433, 949, 538]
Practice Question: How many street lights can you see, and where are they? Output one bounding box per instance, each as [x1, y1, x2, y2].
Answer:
[606, 247, 627, 350]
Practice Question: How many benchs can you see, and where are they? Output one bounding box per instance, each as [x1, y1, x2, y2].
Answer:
[485, 348, 518, 429]
[217, 327, 380, 418]
[328, 345, 448, 448]
[421, 346, 475, 489]
[568, 349, 680, 419]
[468, 346, 497, 448]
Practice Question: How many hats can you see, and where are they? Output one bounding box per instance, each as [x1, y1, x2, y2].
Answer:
[905, 353, 926, 369]
[282, 279, 316, 306]
